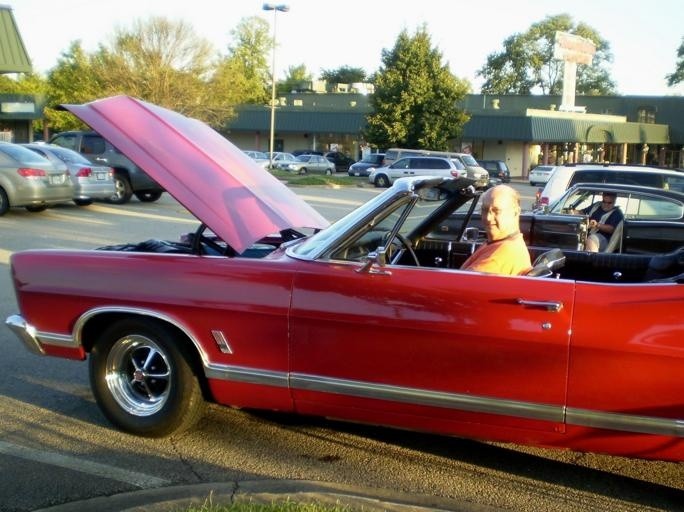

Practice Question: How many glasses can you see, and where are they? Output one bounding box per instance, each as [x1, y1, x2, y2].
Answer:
[602, 200, 616, 204]
[479, 206, 502, 214]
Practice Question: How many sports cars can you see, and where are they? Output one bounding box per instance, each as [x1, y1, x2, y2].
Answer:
[1, 87, 682, 462]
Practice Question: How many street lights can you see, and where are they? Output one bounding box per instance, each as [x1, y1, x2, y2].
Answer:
[261, 0, 291, 171]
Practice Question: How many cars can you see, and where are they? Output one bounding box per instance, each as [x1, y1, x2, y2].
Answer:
[287, 88, 315, 93]
[347, 142, 556, 194]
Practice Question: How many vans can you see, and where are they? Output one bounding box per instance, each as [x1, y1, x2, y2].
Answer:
[528, 160, 684, 219]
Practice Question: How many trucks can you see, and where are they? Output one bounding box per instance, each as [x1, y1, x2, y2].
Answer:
[332, 81, 374, 97]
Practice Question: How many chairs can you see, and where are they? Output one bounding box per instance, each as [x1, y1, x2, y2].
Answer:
[603, 220, 623, 253]
[524, 263, 552, 277]
[533, 248, 566, 267]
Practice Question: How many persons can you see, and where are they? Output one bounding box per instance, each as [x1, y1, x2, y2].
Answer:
[457, 185, 532, 277]
[568, 192, 624, 253]
